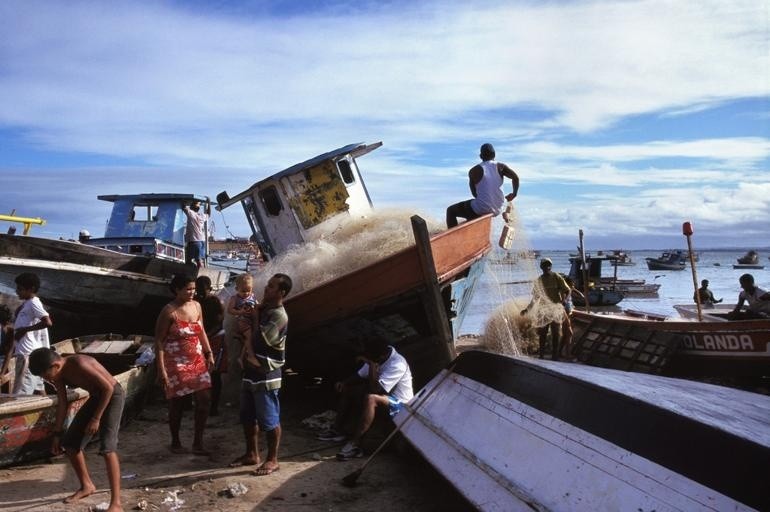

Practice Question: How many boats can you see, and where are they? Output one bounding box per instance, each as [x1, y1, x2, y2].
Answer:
[389, 350, 770, 512]
[644, 253, 686, 270]
[0, 333, 158, 470]
[612, 252, 634, 266]
[563, 259, 662, 305]
[730, 251, 767, 269]
[560, 303, 769, 383]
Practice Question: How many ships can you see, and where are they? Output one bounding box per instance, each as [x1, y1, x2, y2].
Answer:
[217, 142, 490, 391]
[0, 194, 253, 352]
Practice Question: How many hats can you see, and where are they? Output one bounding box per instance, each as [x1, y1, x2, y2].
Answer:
[541, 258, 553, 266]
[481, 143, 495, 153]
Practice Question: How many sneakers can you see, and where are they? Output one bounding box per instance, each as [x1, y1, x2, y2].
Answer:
[336, 442, 363, 460]
[314, 429, 343, 441]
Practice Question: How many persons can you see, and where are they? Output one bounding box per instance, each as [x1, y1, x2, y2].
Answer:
[183, 274, 225, 418]
[181, 195, 212, 267]
[228, 273, 293, 476]
[520, 257, 571, 363]
[154, 273, 216, 455]
[228, 272, 263, 370]
[693, 279, 723, 304]
[446, 143, 519, 230]
[728, 273, 770, 322]
[314, 338, 415, 461]
[12, 274, 52, 397]
[28, 347, 126, 512]
[560, 276, 578, 363]
[0, 304, 15, 384]
